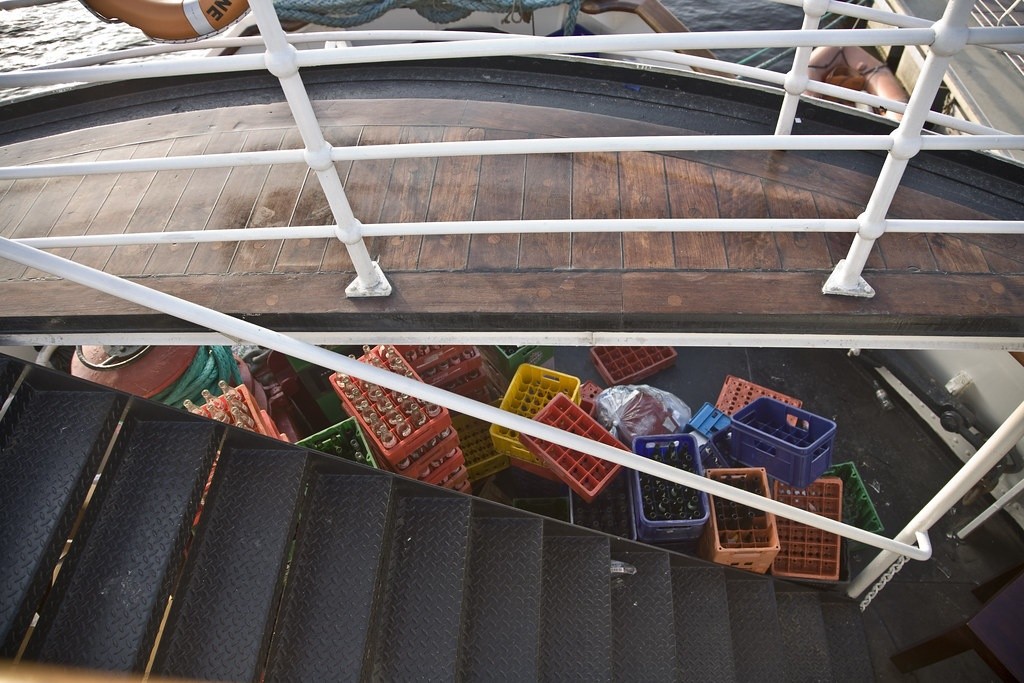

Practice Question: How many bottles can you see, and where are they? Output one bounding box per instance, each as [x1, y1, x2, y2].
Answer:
[505, 348, 515, 355]
[183, 380, 259, 434]
[872, 379, 895, 411]
[575, 443, 762, 539]
[313, 345, 482, 491]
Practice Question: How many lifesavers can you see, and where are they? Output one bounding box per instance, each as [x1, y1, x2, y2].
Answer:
[82, 0, 250, 40]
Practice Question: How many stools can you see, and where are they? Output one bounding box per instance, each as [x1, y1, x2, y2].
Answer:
[890, 567, 1024, 682]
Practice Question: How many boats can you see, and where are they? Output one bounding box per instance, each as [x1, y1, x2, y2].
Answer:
[83, 0, 721, 74]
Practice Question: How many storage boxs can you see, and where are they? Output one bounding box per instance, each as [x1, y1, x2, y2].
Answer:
[182, 345, 884, 582]
